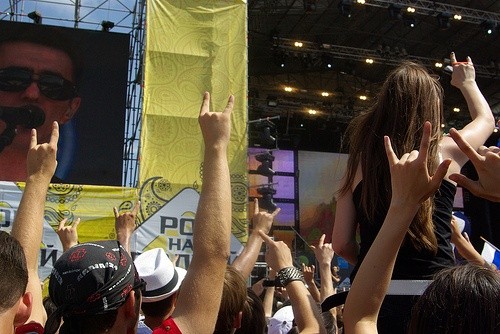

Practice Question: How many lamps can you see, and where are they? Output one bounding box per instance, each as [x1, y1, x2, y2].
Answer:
[101, 20, 114, 31]
[256, 183, 278, 210]
[28, 11, 42, 24]
[257, 119, 277, 150]
[254, 152, 276, 177]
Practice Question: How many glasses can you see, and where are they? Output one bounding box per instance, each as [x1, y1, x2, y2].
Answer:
[131, 278, 147, 292]
[0, 65, 76, 102]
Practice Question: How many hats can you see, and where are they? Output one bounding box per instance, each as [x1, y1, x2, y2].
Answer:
[133, 247, 188, 303]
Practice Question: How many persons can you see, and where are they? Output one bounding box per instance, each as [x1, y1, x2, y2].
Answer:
[0, 59, 500, 334]
[0, 33, 122, 187]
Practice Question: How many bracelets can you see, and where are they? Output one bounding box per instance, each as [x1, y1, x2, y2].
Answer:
[262, 267, 305, 288]
